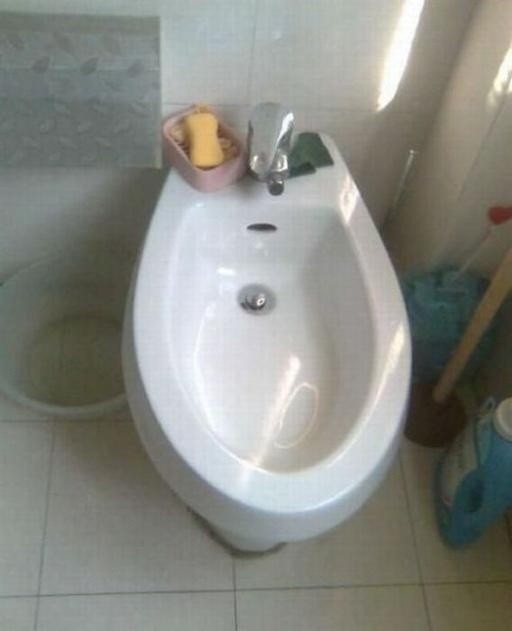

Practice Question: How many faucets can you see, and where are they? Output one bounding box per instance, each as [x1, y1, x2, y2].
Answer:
[242, 100, 296, 196]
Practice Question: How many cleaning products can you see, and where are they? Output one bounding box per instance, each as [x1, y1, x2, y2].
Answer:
[434, 393, 511, 552]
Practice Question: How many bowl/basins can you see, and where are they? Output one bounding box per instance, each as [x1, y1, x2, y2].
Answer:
[0, 246, 134, 422]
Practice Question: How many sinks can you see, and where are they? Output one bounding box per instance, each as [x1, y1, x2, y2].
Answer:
[120, 130, 413, 550]
